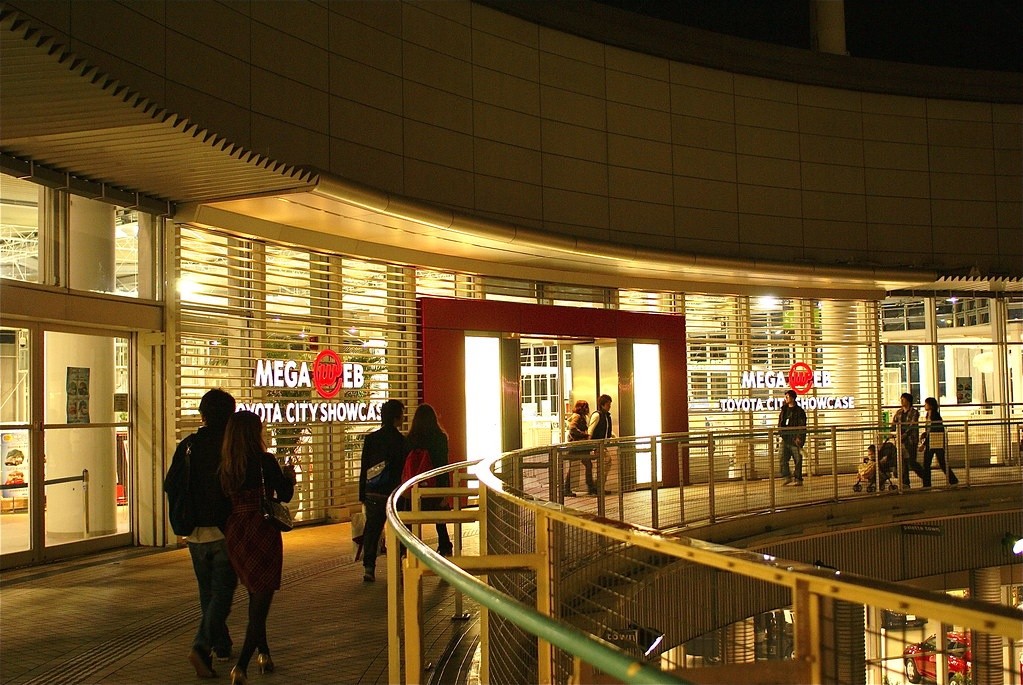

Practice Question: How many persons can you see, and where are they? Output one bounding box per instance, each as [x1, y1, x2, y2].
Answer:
[401, 404, 452, 558]
[588, 395, 618, 495]
[564, 400, 593, 497]
[890, 393, 923, 489]
[218, 412, 294, 685]
[359, 399, 409, 582]
[868, 445, 876, 461]
[164, 389, 239, 677]
[919, 398, 958, 490]
[778, 390, 807, 487]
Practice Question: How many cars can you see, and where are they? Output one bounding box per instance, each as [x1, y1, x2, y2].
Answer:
[904, 632, 972, 685]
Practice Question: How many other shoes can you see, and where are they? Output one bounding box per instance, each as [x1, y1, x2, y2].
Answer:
[949, 481, 961, 488]
[212, 646, 232, 661]
[604, 490, 611, 495]
[188, 645, 219, 678]
[780, 478, 803, 487]
[588, 489, 597, 495]
[564, 490, 576, 497]
[364, 566, 375, 582]
[920, 487, 931, 491]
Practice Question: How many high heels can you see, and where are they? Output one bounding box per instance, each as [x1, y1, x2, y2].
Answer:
[230, 665, 248, 685]
[257, 653, 274, 679]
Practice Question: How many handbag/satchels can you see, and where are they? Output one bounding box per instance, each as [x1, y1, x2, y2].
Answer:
[169, 435, 195, 536]
[351, 504, 385, 564]
[564, 413, 580, 442]
[366, 461, 389, 501]
[259, 453, 293, 532]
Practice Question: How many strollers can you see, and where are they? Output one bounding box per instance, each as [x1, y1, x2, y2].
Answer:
[853, 436, 898, 493]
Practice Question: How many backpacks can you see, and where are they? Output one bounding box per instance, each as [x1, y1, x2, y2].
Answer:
[401, 447, 437, 499]
